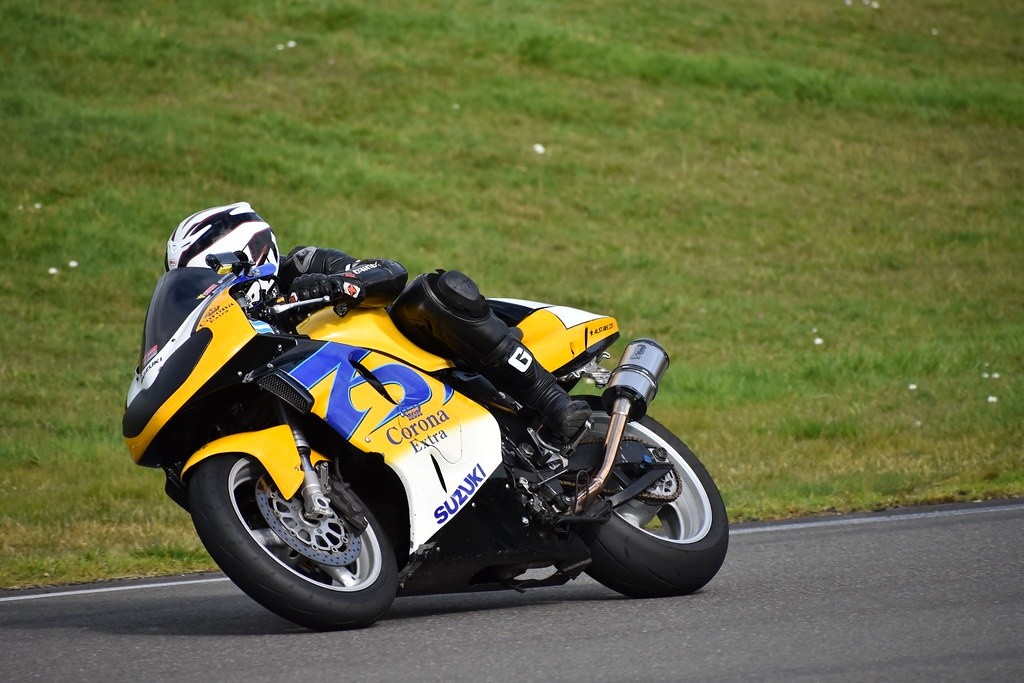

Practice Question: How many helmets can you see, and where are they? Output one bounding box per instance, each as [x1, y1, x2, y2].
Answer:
[163, 201, 280, 308]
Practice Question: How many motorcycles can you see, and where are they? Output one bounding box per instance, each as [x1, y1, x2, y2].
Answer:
[119, 253, 733, 632]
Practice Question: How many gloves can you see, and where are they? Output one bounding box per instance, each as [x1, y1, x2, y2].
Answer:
[287, 274, 344, 307]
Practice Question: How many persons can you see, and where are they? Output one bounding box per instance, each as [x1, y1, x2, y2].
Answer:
[164, 201, 591, 446]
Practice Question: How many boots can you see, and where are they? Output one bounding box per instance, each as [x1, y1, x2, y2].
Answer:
[454, 334, 593, 439]
